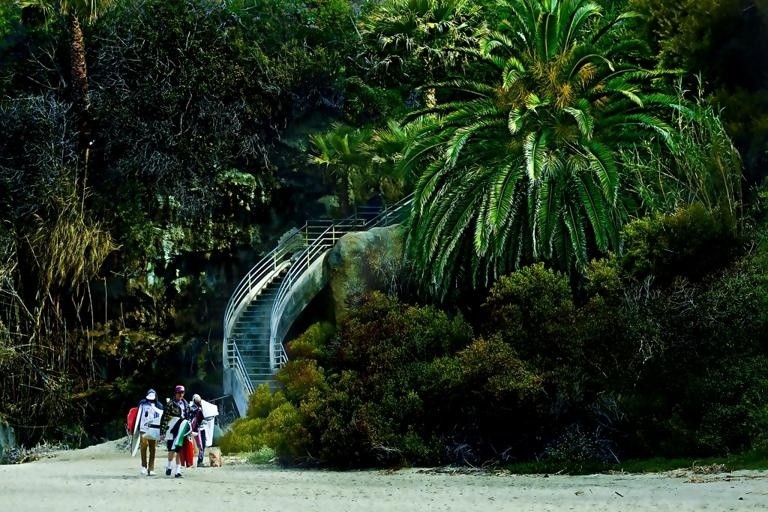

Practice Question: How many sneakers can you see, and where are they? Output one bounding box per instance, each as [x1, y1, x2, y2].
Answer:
[141, 462, 210, 478]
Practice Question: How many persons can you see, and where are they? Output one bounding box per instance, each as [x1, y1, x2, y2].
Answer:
[164, 385, 190, 478]
[137, 389, 164, 475]
[186, 393, 212, 467]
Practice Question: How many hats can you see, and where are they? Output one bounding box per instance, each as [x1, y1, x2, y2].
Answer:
[174, 385, 185, 393]
